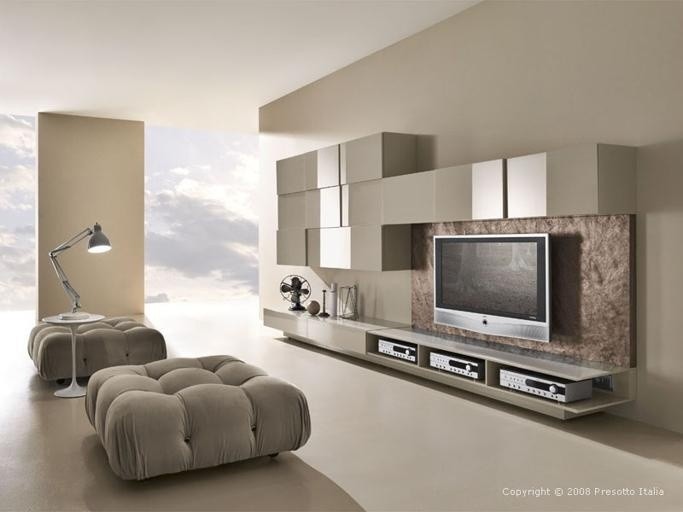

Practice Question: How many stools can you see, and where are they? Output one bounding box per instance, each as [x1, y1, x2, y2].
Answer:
[27, 317, 167, 384]
[84, 354, 311, 481]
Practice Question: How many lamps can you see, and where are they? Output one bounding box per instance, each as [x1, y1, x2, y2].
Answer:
[48, 222, 112, 320]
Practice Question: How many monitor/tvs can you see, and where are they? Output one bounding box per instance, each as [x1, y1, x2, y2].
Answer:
[431, 234, 553, 342]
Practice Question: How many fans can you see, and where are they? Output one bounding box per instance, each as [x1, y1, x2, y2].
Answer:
[279, 275, 311, 312]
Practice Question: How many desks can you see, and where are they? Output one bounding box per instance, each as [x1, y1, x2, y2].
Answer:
[42, 313, 105, 399]
